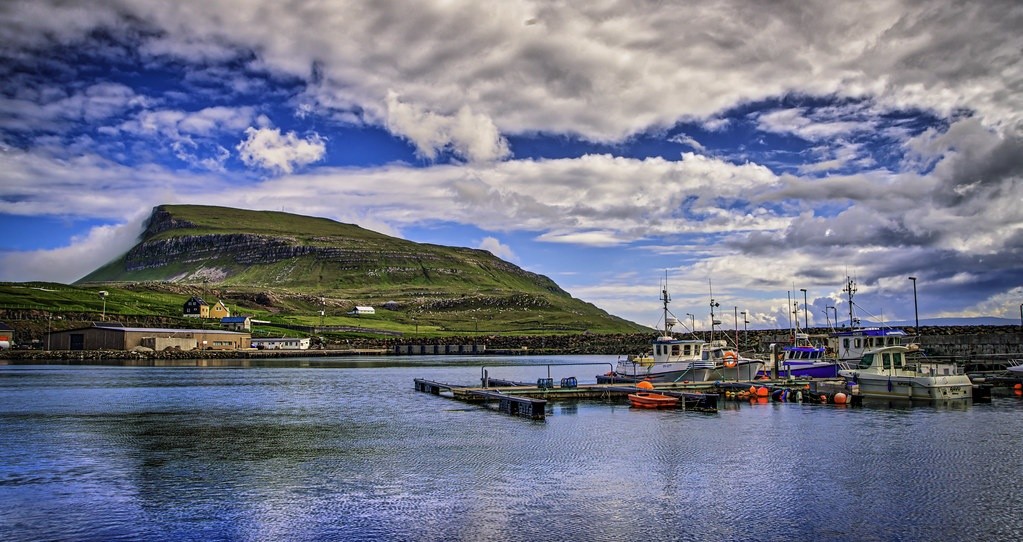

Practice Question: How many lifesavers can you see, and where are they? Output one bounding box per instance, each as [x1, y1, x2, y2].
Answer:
[790, 336, 794, 339]
[800, 346, 813, 348]
[723, 352, 737, 367]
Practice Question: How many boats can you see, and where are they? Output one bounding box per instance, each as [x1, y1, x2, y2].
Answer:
[628, 392, 679, 409]
[596, 262, 1023, 401]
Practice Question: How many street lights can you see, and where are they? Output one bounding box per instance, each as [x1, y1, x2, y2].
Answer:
[687, 313, 694, 331]
[800, 289, 808, 334]
[909, 277, 919, 335]
